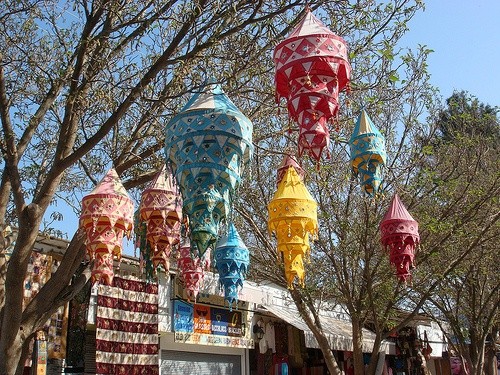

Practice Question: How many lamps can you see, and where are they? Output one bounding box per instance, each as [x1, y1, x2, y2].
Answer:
[255, 325, 265, 339]
